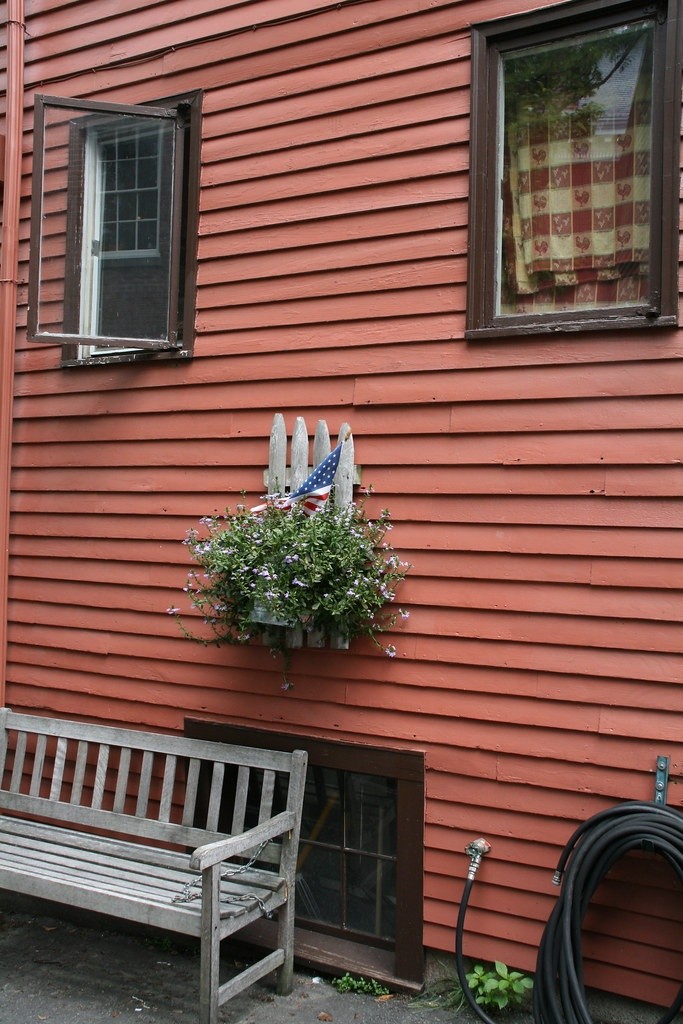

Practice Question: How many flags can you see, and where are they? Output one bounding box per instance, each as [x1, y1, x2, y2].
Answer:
[251, 444, 343, 516]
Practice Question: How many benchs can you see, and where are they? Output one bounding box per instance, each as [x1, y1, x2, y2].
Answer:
[1, 707, 308, 1024]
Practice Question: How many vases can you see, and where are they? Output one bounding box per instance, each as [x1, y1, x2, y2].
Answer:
[250, 588, 302, 630]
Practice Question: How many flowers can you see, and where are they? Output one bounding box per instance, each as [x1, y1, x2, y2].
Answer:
[165, 475, 414, 695]
